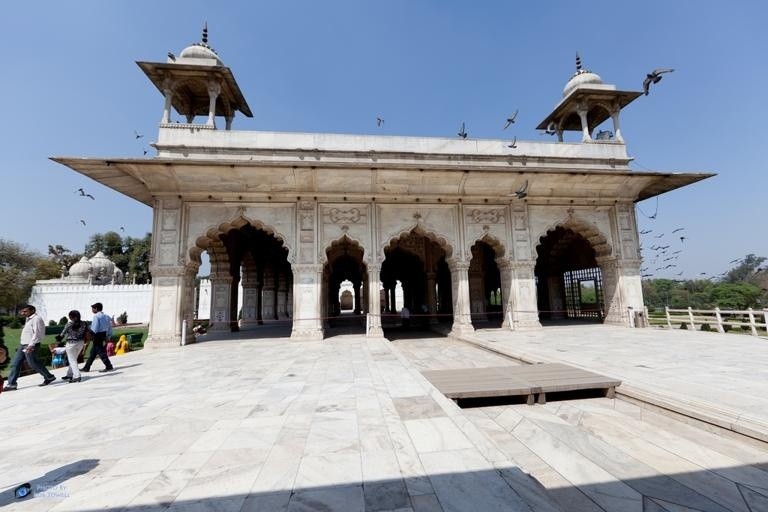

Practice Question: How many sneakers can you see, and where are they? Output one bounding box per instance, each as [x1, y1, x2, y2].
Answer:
[39, 375, 55, 386]
[4, 384, 17, 390]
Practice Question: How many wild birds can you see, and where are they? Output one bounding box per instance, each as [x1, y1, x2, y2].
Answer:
[457, 121, 467, 139]
[538, 122, 555, 136]
[729, 257, 745, 265]
[698, 270, 728, 280]
[642, 68, 675, 96]
[501, 109, 520, 130]
[376, 112, 384, 126]
[77, 188, 96, 200]
[167, 50, 177, 64]
[507, 179, 529, 200]
[634, 226, 688, 278]
[133, 128, 144, 141]
[502, 136, 518, 149]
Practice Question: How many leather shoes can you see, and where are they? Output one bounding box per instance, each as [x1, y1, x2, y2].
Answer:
[79, 367, 89, 372]
[99, 367, 113, 372]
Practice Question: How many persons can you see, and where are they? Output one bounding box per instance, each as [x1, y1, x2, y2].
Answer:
[2, 305, 57, 391]
[78, 303, 115, 373]
[401, 303, 410, 333]
[60, 310, 89, 383]
[47, 333, 130, 369]
[197, 325, 207, 334]
[192, 327, 201, 336]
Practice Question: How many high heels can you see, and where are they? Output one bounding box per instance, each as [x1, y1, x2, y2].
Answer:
[62, 376, 81, 383]
